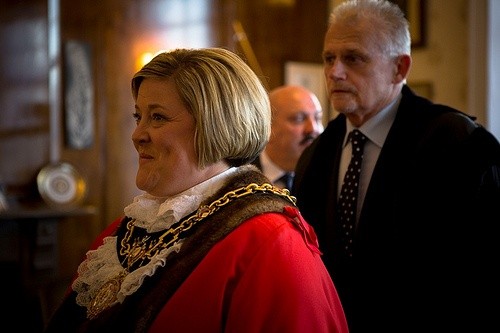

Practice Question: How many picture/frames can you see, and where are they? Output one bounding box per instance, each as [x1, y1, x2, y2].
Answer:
[328, 0, 427, 48]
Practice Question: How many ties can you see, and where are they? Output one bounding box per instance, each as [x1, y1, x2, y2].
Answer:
[282, 175, 294, 194]
[337, 130, 368, 262]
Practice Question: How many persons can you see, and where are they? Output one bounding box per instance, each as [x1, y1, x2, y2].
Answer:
[293, 1, 500, 333]
[253, 85, 323, 193]
[46, 47, 349, 333]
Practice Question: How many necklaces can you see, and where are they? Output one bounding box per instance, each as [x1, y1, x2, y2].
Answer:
[86, 183, 297, 320]
